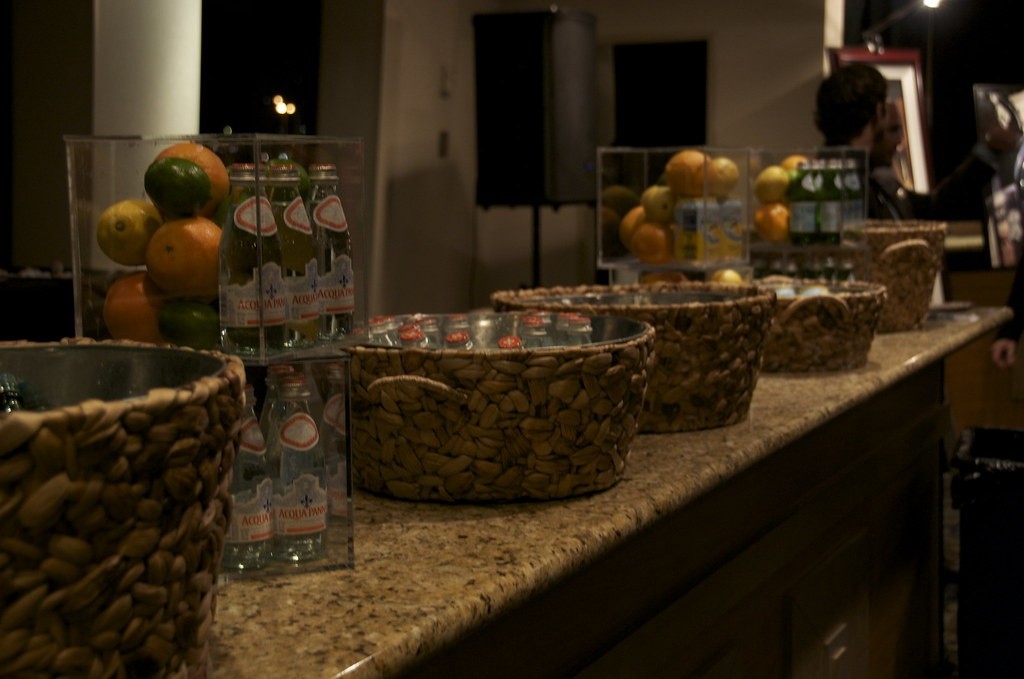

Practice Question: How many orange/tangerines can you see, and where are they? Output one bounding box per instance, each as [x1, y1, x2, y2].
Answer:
[101, 143, 230, 344]
[781, 155, 808, 173]
[619, 150, 715, 265]
[753, 203, 790, 241]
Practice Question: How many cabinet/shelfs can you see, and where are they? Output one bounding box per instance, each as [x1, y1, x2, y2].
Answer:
[391, 360, 949, 679]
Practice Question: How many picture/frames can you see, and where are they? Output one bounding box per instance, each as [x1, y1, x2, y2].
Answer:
[831, 46, 938, 197]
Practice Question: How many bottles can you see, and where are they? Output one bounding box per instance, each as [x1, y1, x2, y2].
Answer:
[752, 253, 857, 282]
[223, 364, 349, 570]
[788, 157, 863, 246]
[988, 174, 1018, 270]
[1, 374, 24, 413]
[216, 163, 355, 357]
[365, 308, 594, 350]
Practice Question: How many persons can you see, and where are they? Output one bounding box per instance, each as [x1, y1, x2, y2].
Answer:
[991, 251, 1024, 369]
[788, 62, 1022, 220]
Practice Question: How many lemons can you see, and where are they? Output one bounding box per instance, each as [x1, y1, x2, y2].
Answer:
[157, 298, 220, 349]
[96, 200, 165, 265]
[710, 268, 744, 285]
[641, 185, 676, 223]
[709, 157, 740, 199]
[751, 165, 790, 200]
[145, 157, 214, 218]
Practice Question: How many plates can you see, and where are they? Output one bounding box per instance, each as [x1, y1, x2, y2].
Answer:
[928, 300, 974, 312]
